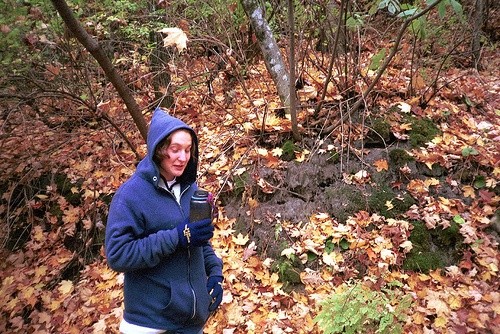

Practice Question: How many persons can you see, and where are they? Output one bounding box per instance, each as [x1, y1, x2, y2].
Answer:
[104, 107, 225, 334]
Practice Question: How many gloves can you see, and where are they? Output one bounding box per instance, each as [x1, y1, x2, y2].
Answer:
[207, 276, 224, 311]
[176, 216, 215, 249]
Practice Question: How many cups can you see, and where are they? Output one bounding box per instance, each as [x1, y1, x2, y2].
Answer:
[190, 190, 211, 223]
